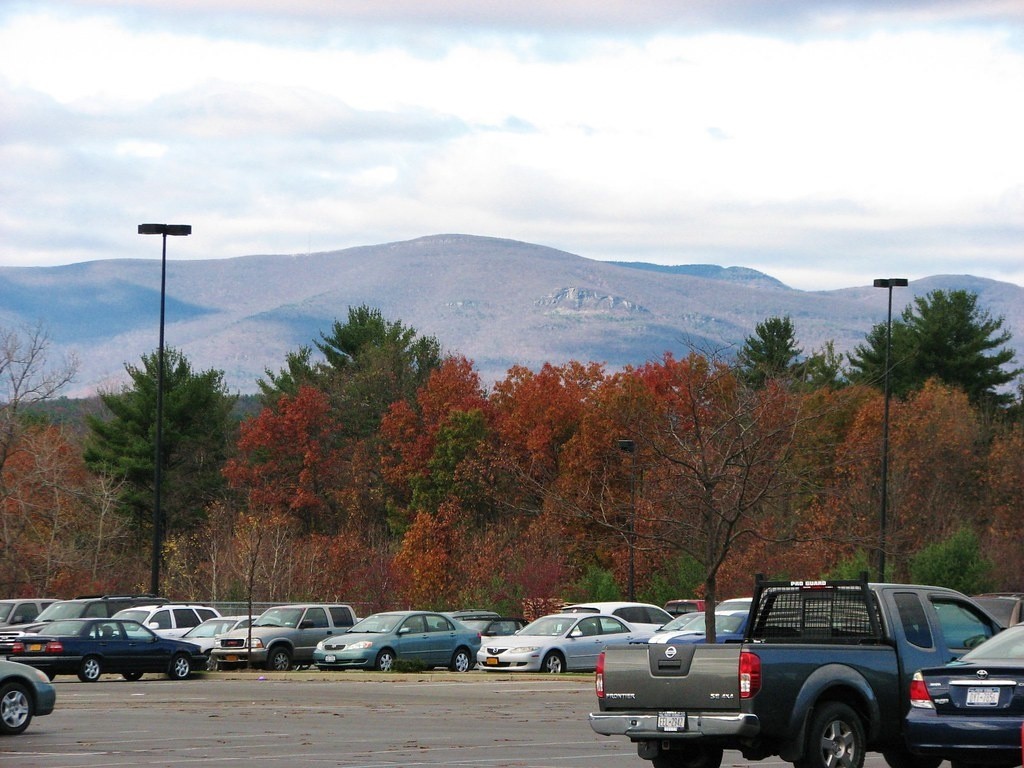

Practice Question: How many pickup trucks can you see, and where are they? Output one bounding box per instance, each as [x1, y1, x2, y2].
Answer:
[211, 604, 360, 671]
[588, 569, 1009, 768]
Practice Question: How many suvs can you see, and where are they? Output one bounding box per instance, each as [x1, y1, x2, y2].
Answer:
[439, 609, 504, 622]
[0, 595, 172, 654]
[89, 604, 223, 639]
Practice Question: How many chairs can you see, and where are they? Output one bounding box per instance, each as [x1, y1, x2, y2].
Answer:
[103, 627, 114, 638]
[437, 621, 447, 630]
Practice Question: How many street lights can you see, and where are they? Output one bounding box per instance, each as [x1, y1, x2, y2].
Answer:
[140, 222, 195, 595]
[874, 279, 908, 581]
[618, 439, 636, 602]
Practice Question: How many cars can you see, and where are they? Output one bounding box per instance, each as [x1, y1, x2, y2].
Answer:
[966, 592, 1024, 628]
[629, 610, 751, 645]
[312, 611, 483, 672]
[0, 600, 64, 627]
[459, 617, 531, 636]
[661, 600, 720, 618]
[904, 621, 1024, 768]
[179, 616, 282, 671]
[714, 598, 754, 612]
[0, 619, 209, 736]
[476, 613, 656, 673]
[556, 601, 685, 638]
